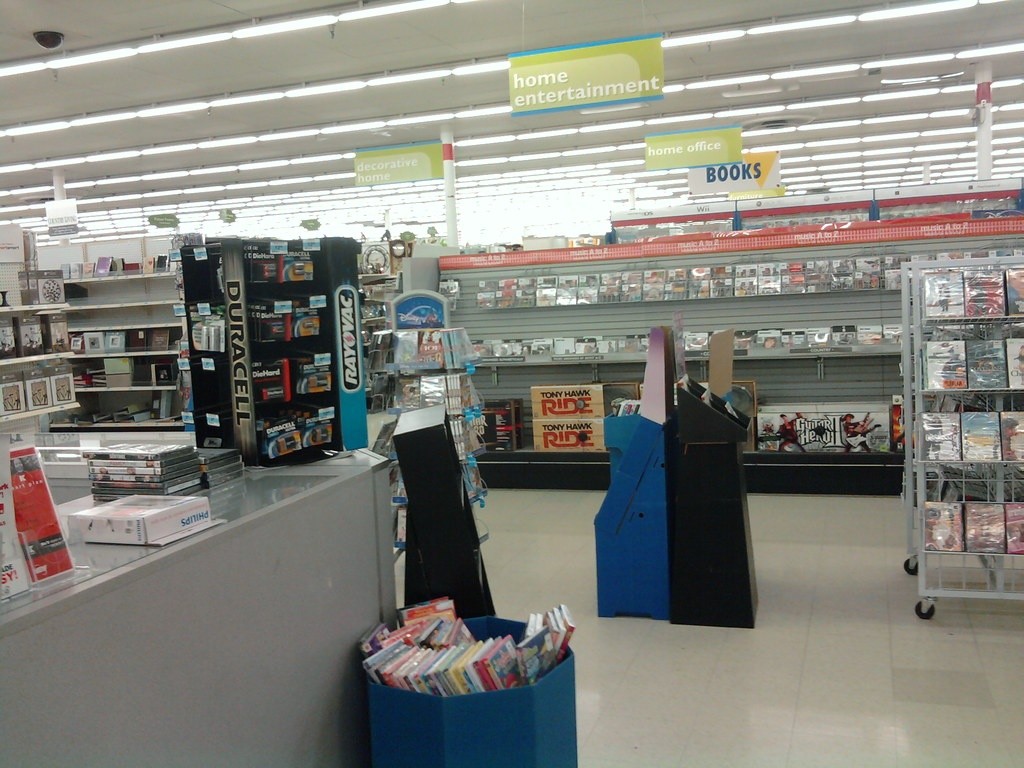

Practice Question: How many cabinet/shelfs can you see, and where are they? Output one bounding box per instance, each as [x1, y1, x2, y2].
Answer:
[0, 223, 79, 467]
[881, 217, 1023, 498]
[366, 321, 491, 564]
[434, 217, 882, 498]
[75, 202, 460, 475]
[898, 257, 1024, 558]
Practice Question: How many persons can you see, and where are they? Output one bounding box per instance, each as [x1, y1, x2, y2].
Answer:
[917, 271, 1024, 556]
[472, 324, 904, 358]
[777, 412, 807, 453]
[475, 248, 1024, 315]
[841, 414, 872, 452]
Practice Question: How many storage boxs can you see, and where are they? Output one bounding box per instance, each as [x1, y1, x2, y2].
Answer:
[66, 494, 224, 547]
[0, 224, 76, 415]
[470, 376, 918, 451]
